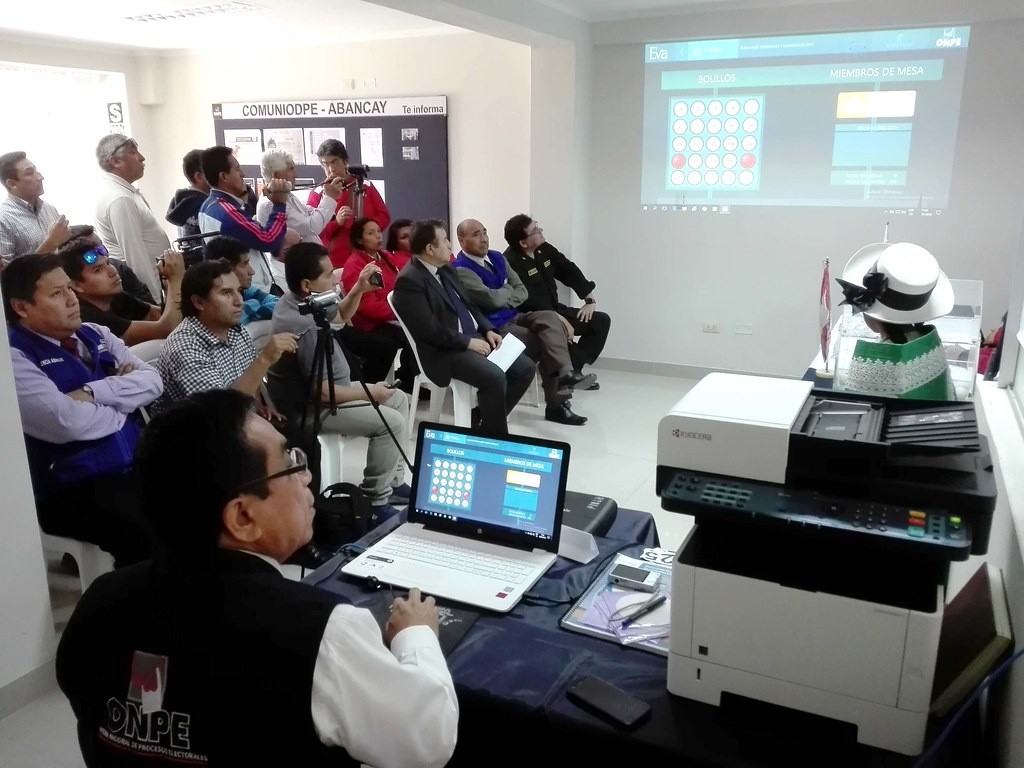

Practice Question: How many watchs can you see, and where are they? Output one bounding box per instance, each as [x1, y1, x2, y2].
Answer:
[585, 298, 596, 304]
[83, 385, 94, 400]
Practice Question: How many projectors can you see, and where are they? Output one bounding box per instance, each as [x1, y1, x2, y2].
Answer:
[562, 489, 618, 538]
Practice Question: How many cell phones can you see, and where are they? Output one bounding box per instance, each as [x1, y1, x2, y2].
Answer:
[369, 272, 384, 288]
[566, 673, 652, 732]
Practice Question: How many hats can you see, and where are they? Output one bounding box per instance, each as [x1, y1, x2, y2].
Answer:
[835, 242, 955, 324]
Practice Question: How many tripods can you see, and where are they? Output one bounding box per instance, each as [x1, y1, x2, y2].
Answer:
[295, 312, 415, 476]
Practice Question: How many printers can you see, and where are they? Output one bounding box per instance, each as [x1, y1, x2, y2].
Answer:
[652, 372, 998, 756]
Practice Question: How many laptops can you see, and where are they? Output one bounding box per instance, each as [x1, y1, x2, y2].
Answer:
[342, 421, 571, 614]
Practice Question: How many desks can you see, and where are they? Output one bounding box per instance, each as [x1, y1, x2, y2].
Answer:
[276, 493, 1024, 768]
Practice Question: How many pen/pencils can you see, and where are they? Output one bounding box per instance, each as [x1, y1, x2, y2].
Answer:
[622, 595, 667, 627]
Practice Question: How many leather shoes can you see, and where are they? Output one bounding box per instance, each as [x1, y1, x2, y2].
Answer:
[545, 403, 588, 425]
[557, 373, 598, 396]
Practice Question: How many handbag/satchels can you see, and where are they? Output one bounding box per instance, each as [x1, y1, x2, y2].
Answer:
[284, 479, 381, 551]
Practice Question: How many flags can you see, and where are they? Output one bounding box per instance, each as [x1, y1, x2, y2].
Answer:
[818, 263, 831, 361]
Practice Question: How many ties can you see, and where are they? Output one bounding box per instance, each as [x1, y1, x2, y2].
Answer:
[60, 336, 95, 375]
[436, 268, 477, 338]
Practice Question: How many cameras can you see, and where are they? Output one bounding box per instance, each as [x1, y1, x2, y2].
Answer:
[298, 290, 337, 315]
[608, 563, 662, 592]
[346, 164, 370, 176]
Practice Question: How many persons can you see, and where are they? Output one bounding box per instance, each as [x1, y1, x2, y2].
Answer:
[166, 146, 288, 293]
[451, 213, 611, 426]
[148, 259, 321, 499]
[60, 224, 184, 346]
[57, 387, 459, 768]
[205, 237, 281, 327]
[835, 241, 958, 402]
[340, 217, 456, 399]
[393, 219, 536, 437]
[0, 253, 164, 569]
[95, 133, 171, 308]
[304, 140, 390, 268]
[271, 242, 409, 521]
[0, 149, 72, 265]
[254, 149, 343, 252]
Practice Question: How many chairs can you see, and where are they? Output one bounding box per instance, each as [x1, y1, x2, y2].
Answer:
[42, 291, 538, 592]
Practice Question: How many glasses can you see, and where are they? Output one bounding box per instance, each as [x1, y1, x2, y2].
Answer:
[527, 220, 540, 237]
[69, 245, 108, 264]
[226, 447, 308, 502]
[318, 156, 341, 169]
[110, 138, 139, 156]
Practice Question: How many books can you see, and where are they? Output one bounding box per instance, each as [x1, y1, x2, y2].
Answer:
[486, 333, 526, 374]
[559, 551, 672, 657]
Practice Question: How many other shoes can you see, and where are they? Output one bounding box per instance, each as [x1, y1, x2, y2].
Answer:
[388, 482, 411, 503]
[572, 372, 599, 391]
[471, 406, 482, 430]
[370, 504, 401, 531]
